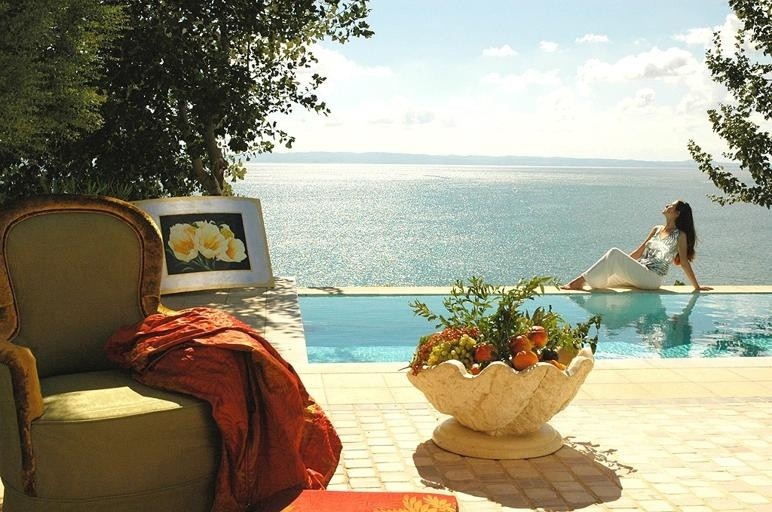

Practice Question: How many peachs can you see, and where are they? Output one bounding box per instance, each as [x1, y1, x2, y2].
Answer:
[473, 323, 547, 374]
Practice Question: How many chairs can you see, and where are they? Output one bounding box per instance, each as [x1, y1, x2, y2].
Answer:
[0, 193, 244, 512]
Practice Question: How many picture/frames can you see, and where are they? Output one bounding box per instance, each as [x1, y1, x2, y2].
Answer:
[126, 196, 275, 295]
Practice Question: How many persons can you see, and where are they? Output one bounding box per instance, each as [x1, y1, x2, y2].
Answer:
[562, 200, 716, 291]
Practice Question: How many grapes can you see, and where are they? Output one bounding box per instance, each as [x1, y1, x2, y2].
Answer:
[428, 333, 477, 368]
